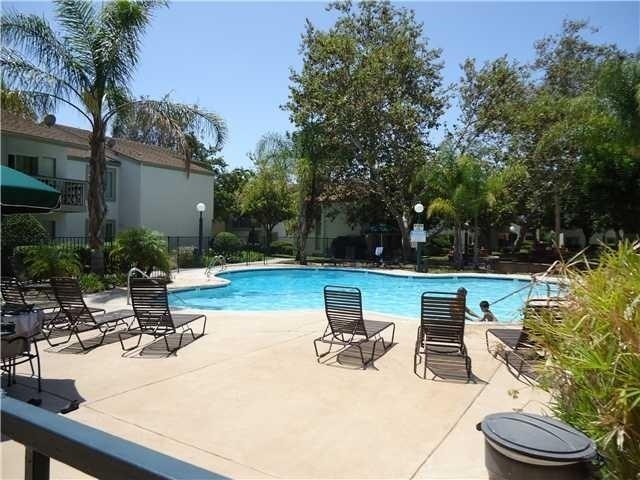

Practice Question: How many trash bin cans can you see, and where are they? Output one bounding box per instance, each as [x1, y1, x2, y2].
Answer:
[476, 412, 598, 480]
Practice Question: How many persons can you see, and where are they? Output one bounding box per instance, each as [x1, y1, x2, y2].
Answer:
[450, 287, 481, 321]
[474, 301, 498, 322]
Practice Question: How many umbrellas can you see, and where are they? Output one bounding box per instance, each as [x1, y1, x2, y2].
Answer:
[365, 224, 399, 247]
[0, 165, 62, 216]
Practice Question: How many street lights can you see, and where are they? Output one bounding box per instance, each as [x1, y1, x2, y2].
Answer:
[413, 203, 424, 264]
[196, 202, 206, 250]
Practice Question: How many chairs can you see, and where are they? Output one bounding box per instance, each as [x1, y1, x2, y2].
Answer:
[0, 275, 206, 351]
[485, 298, 568, 378]
[413, 292, 472, 381]
[313, 285, 395, 368]
